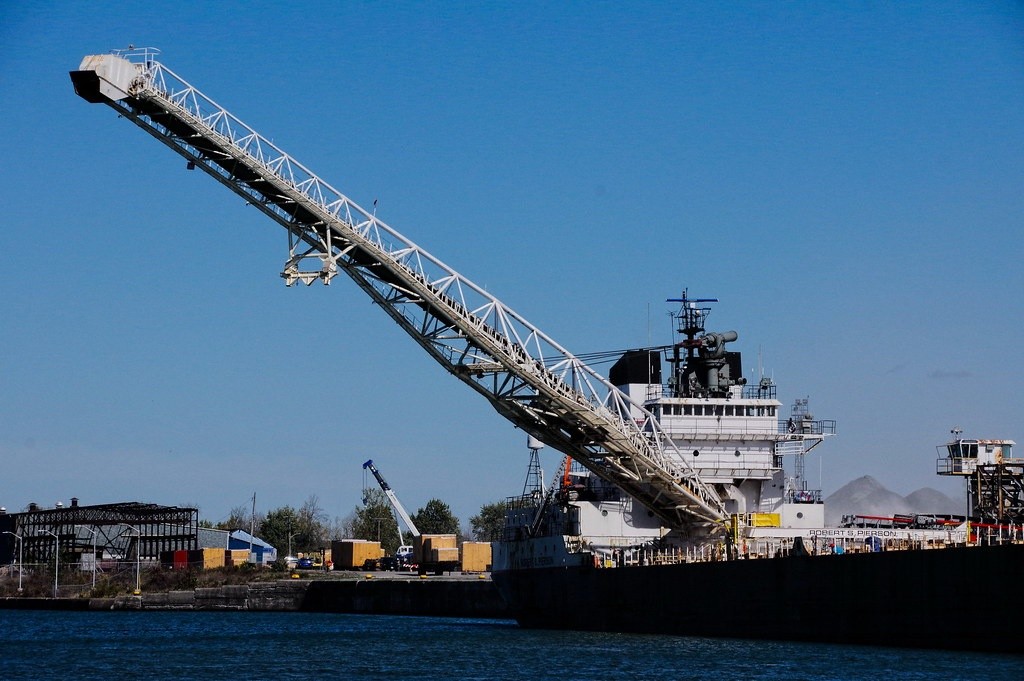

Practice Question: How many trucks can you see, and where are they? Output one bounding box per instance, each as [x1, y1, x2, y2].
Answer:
[394, 545, 413, 566]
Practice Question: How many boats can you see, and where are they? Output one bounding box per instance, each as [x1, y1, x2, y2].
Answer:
[67, 43, 1024, 656]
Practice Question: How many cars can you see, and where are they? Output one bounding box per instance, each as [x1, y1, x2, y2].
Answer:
[284, 556, 298, 569]
[298, 557, 315, 570]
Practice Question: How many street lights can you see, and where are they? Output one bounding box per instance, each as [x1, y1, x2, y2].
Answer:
[38, 529, 58, 598]
[73, 525, 97, 589]
[119, 522, 141, 595]
[3, 532, 23, 591]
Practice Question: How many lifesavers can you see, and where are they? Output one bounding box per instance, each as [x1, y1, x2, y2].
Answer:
[800, 491, 811, 501]
